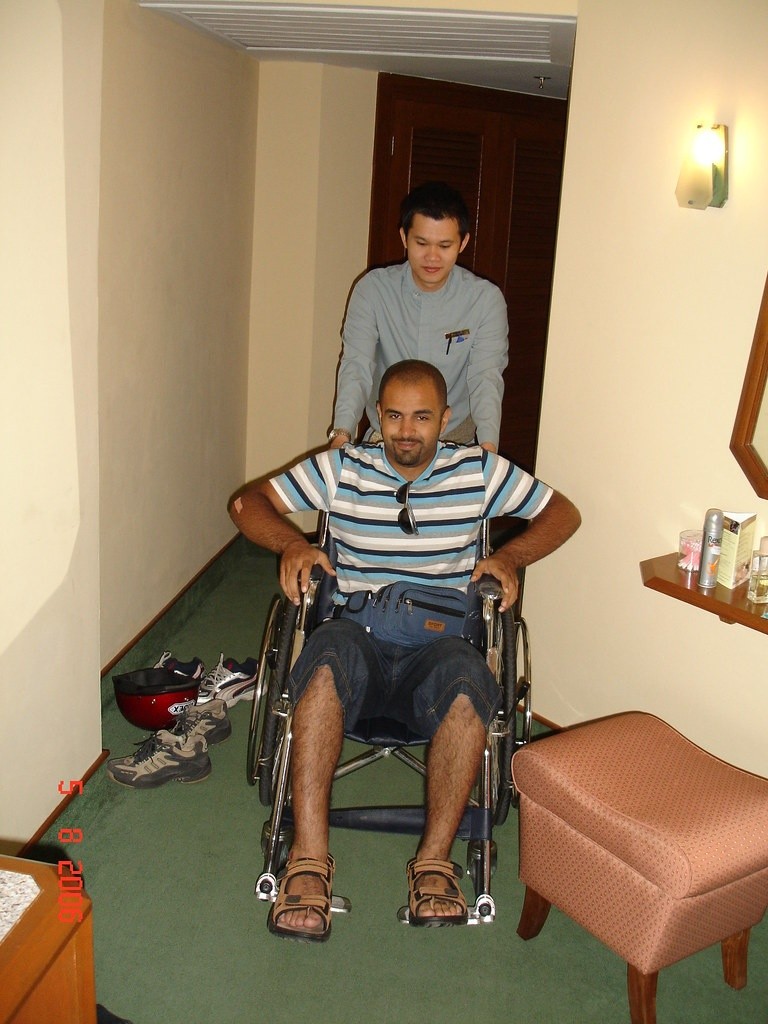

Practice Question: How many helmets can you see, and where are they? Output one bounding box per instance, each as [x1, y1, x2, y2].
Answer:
[111, 667, 202, 730]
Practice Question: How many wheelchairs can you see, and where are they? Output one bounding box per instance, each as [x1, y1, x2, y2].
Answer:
[242, 429, 536, 929]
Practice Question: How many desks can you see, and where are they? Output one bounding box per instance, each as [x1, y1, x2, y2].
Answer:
[0, 854, 96, 1024]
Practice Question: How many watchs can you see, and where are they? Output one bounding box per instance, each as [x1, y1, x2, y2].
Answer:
[329, 427, 352, 444]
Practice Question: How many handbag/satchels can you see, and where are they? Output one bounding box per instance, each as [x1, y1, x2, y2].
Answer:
[332, 581, 468, 647]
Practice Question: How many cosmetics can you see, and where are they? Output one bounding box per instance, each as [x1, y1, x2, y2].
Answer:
[697, 508, 768, 604]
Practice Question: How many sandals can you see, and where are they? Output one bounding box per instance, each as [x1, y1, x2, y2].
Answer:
[266, 853, 335, 941]
[406, 857, 469, 928]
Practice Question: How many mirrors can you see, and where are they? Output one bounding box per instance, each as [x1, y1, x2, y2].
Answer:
[728, 271, 768, 500]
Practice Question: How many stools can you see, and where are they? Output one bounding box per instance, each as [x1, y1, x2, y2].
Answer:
[510, 711, 768, 1024]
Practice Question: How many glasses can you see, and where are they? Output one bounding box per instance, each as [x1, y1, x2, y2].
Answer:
[395, 481, 420, 535]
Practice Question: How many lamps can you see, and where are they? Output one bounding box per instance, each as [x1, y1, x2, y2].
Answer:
[674, 121, 729, 212]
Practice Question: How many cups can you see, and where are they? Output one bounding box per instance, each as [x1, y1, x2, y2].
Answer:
[679, 530, 703, 573]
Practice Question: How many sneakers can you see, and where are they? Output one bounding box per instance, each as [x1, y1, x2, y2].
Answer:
[168, 699, 232, 745]
[195, 651, 268, 709]
[153, 651, 206, 679]
[107, 729, 214, 790]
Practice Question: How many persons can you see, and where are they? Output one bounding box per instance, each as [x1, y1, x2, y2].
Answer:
[228, 359, 582, 943]
[326, 182, 510, 455]
